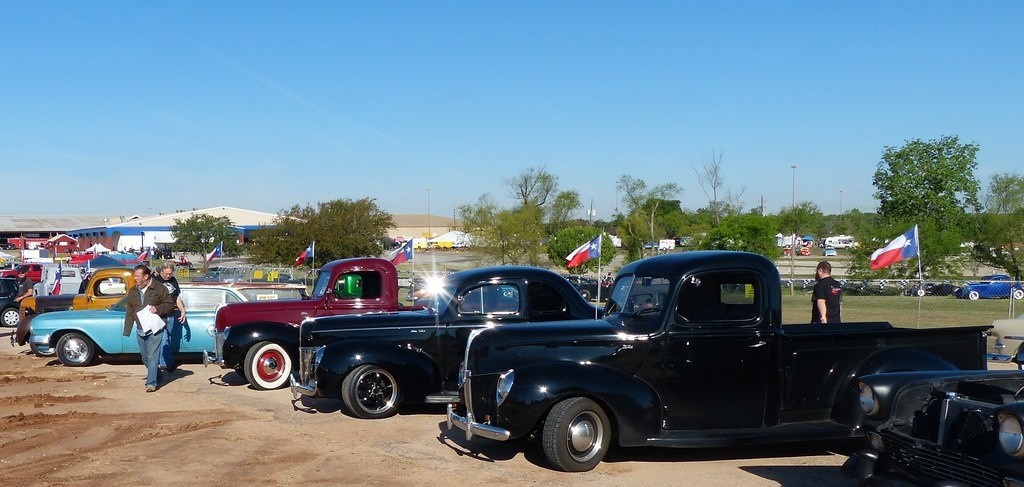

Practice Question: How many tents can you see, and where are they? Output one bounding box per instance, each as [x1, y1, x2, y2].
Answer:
[85, 243, 110, 258]
[428, 231, 488, 248]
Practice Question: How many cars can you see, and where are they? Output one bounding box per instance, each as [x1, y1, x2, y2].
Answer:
[288, 264, 604, 420]
[27, 285, 253, 368]
[904, 282, 959, 297]
[985, 313, 1024, 363]
[955, 274, 1024, 301]
[405, 275, 518, 305]
[0, 266, 140, 358]
[783, 237, 855, 256]
[561, 272, 671, 312]
[838, 342, 1024, 487]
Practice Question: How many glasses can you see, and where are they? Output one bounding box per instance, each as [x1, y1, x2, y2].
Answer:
[19, 272, 25, 274]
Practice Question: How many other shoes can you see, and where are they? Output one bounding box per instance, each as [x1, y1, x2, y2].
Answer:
[146, 384, 156, 392]
[157, 375, 164, 383]
[160, 366, 176, 372]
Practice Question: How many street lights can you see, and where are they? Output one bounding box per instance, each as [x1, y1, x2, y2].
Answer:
[791, 164, 797, 209]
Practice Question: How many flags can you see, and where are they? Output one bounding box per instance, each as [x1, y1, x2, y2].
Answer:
[51, 262, 62, 295]
[565, 234, 599, 268]
[135, 250, 148, 263]
[390, 239, 413, 265]
[870, 226, 918, 271]
[295, 245, 312, 268]
[206, 244, 221, 265]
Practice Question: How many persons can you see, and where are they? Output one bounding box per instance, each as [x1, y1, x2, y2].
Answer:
[90, 241, 93, 247]
[174, 256, 192, 267]
[124, 265, 175, 391]
[12, 268, 33, 317]
[69, 248, 72, 255]
[601, 272, 615, 300]
[811, 261, 842, 324]
[123, 244, 173, 260]
[78, 269, 93, 294]
[151, 263, 185, 372]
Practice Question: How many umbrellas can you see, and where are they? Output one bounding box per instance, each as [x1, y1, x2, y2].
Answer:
[53, 247, 57, 263]
[81, 255, 126, 268]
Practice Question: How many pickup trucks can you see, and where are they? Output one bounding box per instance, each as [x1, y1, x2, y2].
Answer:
[202, 257, 520, 391]
[446, 249, 912, 473]
